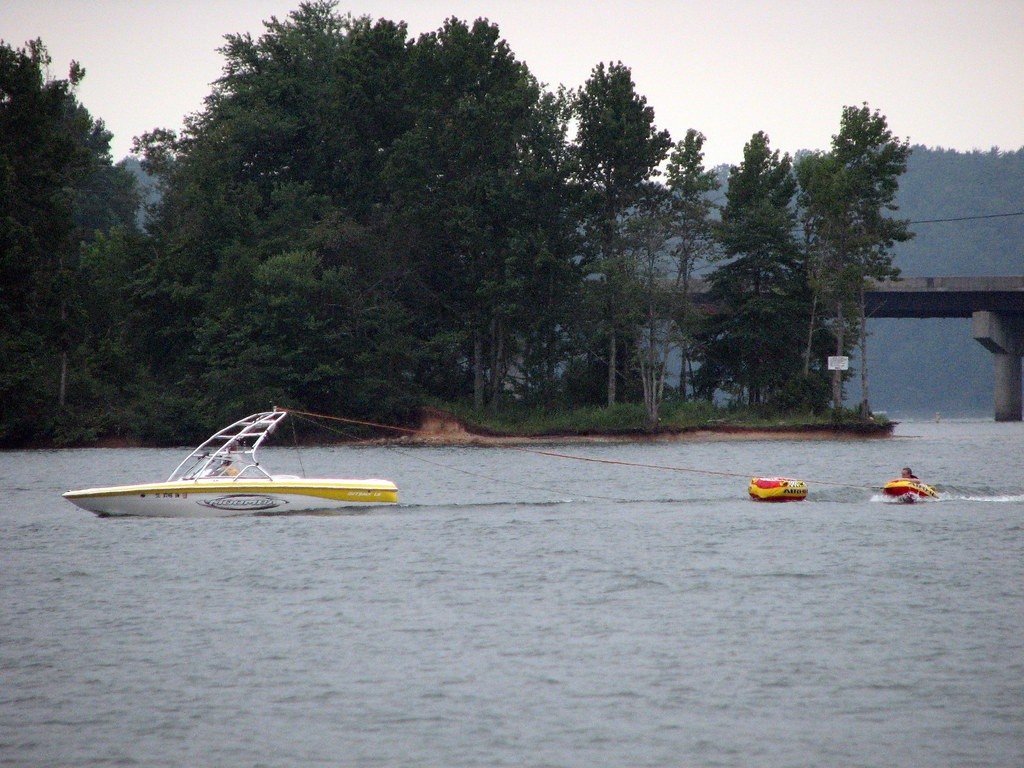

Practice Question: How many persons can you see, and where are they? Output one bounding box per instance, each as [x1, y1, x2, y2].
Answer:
[223, 457, 238, 475]
[901, 468, 920, 483]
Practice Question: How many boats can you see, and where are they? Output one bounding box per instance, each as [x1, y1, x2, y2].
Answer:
[745, 475, 808, 504]
[881, 479, 940, 503]
[60, 409, 398, 520]
